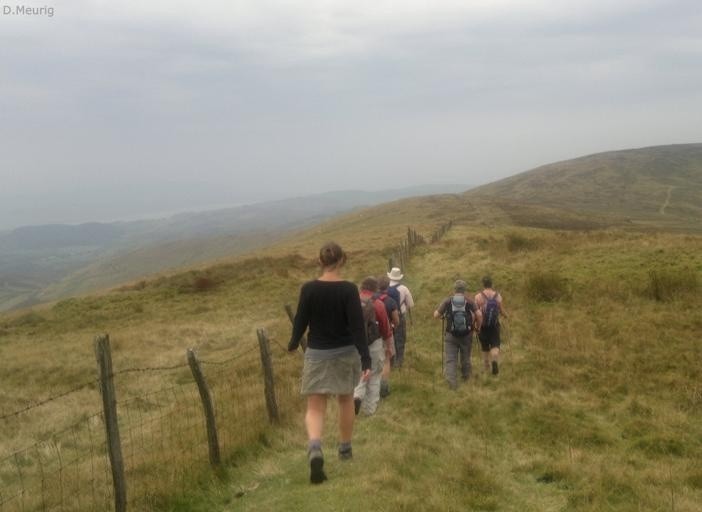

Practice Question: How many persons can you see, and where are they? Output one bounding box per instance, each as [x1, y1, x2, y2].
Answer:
[353, 267, 415, 418]
[433, 279, 483, 391]
[474, 277, 508, 375]
[288, 242, 371, 483]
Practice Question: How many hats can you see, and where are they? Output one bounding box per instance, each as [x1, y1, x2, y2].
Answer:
[387, 267, 404, 281]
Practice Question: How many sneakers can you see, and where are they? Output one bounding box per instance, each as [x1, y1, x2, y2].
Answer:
[354, 393, 361, 414]
[492, 360, 499, 375]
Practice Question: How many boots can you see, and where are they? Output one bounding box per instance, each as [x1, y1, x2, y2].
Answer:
[380, 383, 390, 397]
[339, 447, 351, 460]
[308, 446, 326, 484]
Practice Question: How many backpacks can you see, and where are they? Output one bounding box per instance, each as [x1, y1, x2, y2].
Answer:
[447, 297, 474, 336]
[387, 283, 405, 318]
[360, 292, 382, 344]
[480, 290, 501, 328]
